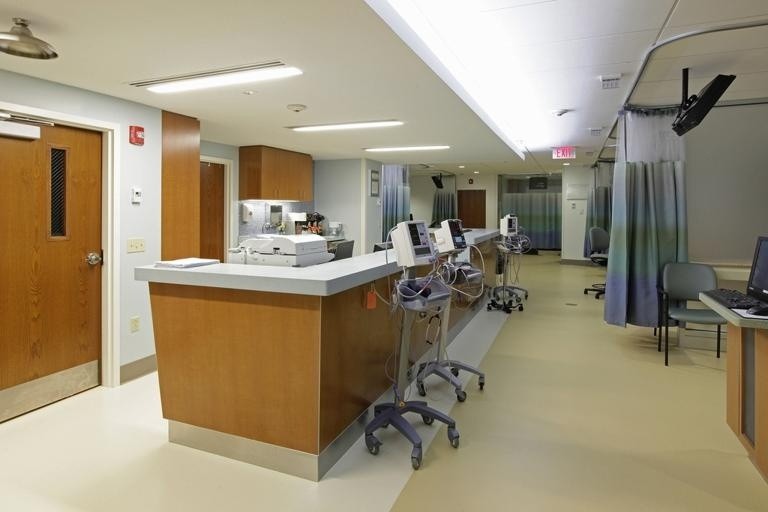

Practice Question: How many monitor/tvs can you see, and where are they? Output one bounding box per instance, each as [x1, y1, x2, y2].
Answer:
[390, 220, 437, 267]
[434, 219, 467, 254]
[746, 236, 768, 304]
[432, 176, 443, 188]
[529, 177, 547, 189]
[672, 74, 736, 136]
[499, 216, 518, 237]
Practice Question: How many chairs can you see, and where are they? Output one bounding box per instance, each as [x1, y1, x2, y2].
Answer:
[658, 263, 727, 366]
[590, 227, 609, 264]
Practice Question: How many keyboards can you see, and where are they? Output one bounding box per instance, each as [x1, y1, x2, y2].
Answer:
[703, 288, 760, 308]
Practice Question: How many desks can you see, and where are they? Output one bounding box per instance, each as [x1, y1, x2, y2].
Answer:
[698, 290, 768, 483]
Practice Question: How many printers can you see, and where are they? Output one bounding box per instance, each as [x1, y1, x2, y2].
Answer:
[227, 232, 335, 267]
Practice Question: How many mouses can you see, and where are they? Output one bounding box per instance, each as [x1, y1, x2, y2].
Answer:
[746, 306, 768, 316]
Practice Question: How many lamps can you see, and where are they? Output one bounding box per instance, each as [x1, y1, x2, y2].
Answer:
[1, 17, 59, 59]
